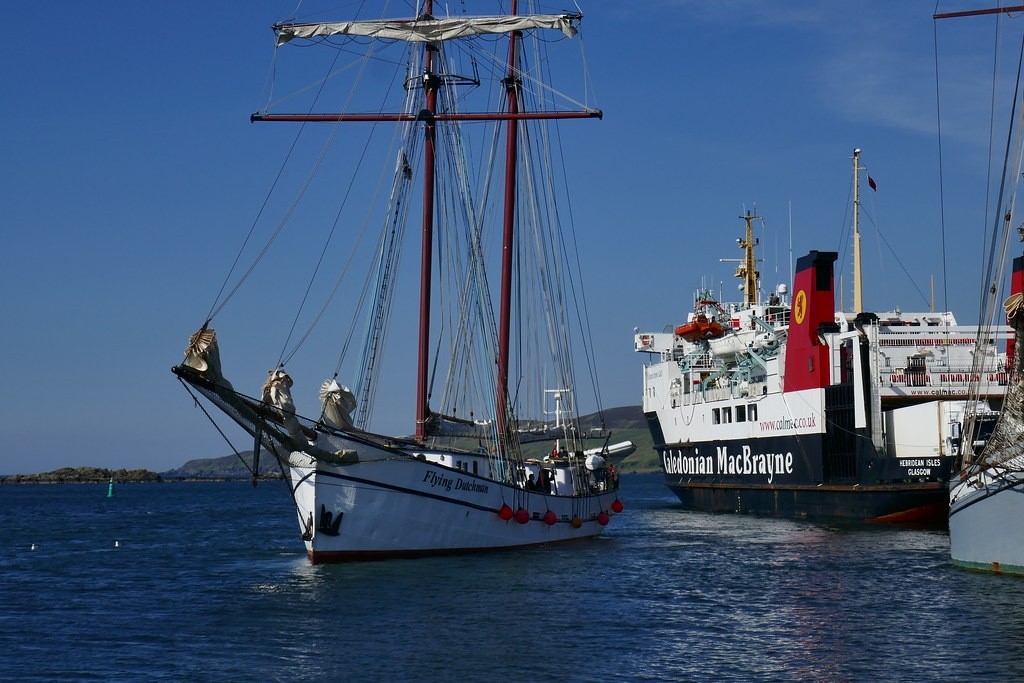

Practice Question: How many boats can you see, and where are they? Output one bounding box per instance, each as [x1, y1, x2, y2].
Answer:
[543, 438, 637, 469]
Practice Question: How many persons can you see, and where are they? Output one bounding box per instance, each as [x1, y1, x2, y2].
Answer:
[527, 474, 536, 491]
[552, 443, 568, 466]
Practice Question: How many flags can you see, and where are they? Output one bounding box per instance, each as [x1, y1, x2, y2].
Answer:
[867, 174, 876, 192]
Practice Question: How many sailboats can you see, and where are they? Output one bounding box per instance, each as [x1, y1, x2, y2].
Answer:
[634, 0, 1024, 531]
[948, 121, 1023, 581]
[160, 0, 625, 565]
